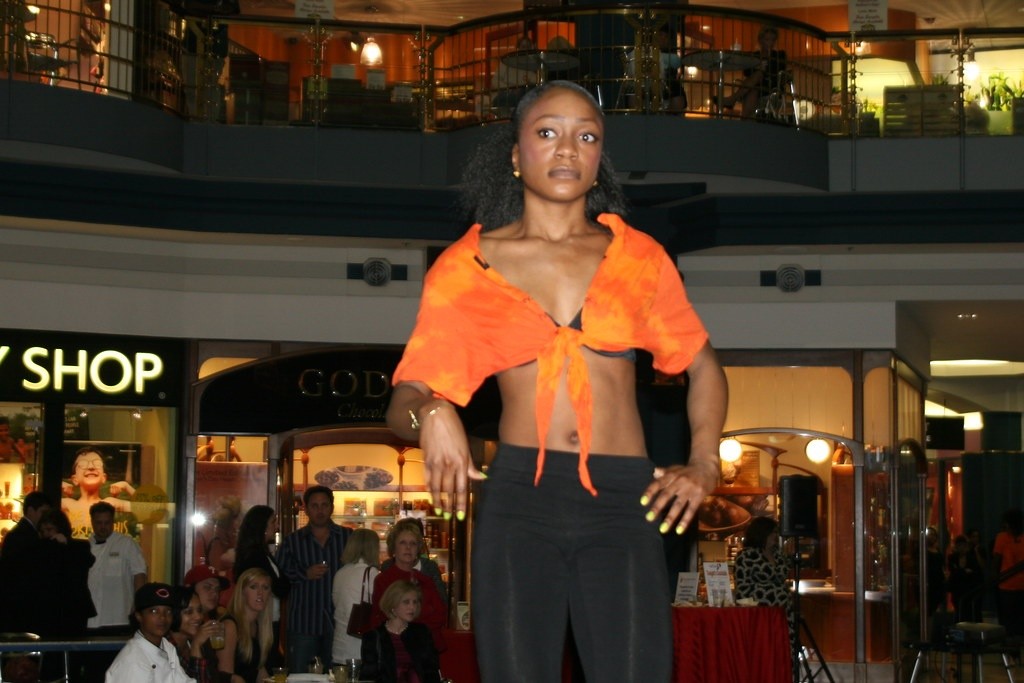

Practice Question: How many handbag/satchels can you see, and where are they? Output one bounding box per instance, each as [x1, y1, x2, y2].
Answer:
[346, 566, 377, 639]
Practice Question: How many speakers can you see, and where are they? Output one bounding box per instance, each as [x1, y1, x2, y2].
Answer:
[778, 475, 819, 538]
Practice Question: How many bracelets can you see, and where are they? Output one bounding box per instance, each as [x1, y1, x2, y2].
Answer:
[407, 405, 455, 430]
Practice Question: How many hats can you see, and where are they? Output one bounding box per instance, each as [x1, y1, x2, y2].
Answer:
[185, 564, 230, 590]
[134, 582, 178, 610]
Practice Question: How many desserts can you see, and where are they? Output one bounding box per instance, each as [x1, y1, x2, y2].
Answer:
[699, 494, 741, 526]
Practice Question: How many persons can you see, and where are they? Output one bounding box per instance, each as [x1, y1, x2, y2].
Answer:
[925, 506, 1024, 668]
[106, 485, 447, 683]
[387, 80, 729, 683]
[61, 449, 137, 539]
[732, 516, 800, 683]
[711, 25, 789, 120]
[631, 28, 688, 116]
[0, 491, 99, 683]
[79, 501, 147, 683]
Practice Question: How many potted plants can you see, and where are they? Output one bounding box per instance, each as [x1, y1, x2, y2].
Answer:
[991, 72, 1024, 136]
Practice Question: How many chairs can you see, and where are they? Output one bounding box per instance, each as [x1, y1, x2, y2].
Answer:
[906, 570, 1015, 683]
[732, 66, 794, 124]
[611, 48, 665, 117]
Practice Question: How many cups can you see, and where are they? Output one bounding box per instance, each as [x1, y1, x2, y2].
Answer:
[209, 623, 224, 649]
[320, 560, 328, 564]
[404, 501, 412, 510]
[346, 658, 362, 683]
[273, 668, 287, 683]
[333, 666, 349, 683]
[311, 657, 323, 674]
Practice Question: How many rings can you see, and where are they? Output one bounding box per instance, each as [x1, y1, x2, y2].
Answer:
[212, 621, 216, 626]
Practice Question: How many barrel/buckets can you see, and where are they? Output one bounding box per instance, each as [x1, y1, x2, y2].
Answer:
[394, 81, 412, 102]
[331, 63, 356, 79]
[366, 70, 386, 90]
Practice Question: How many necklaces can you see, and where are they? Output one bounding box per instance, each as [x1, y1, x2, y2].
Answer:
[386, 620, 408, 635]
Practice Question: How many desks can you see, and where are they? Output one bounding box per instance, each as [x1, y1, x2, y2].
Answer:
[440, 597, 794, 683]
[0, 635, 132, 683]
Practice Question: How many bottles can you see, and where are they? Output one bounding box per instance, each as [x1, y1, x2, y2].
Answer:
[426, 522, 432, 538]
[761, 56, 768, 72]
[433, 530, 439, 548]
[24, 464, 39, 496]
[24, 417, 40, 432]
[441, 532, 447, 548]
[64, 415, 79, 434]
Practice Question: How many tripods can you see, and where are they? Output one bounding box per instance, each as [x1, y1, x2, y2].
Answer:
[789, 538, 835, 683]
